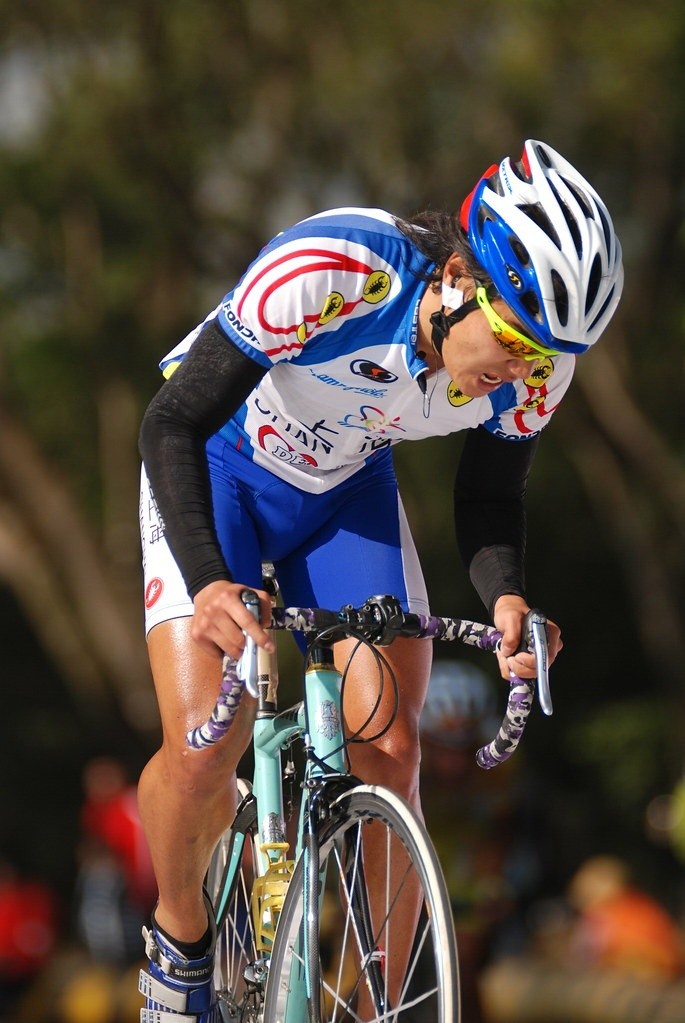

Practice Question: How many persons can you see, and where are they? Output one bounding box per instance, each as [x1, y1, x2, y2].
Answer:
[137, 141, 625, 1022]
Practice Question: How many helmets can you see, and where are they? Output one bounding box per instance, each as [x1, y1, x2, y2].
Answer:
[459, 138, 625, 355]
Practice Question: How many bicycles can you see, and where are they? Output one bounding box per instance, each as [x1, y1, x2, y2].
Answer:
[182, 587, 557, 1023]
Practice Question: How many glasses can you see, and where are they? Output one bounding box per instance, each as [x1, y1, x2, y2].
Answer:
[476, 287, 564, 362]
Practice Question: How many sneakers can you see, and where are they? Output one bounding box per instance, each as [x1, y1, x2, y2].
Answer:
[138, 887, 217, 1023]
